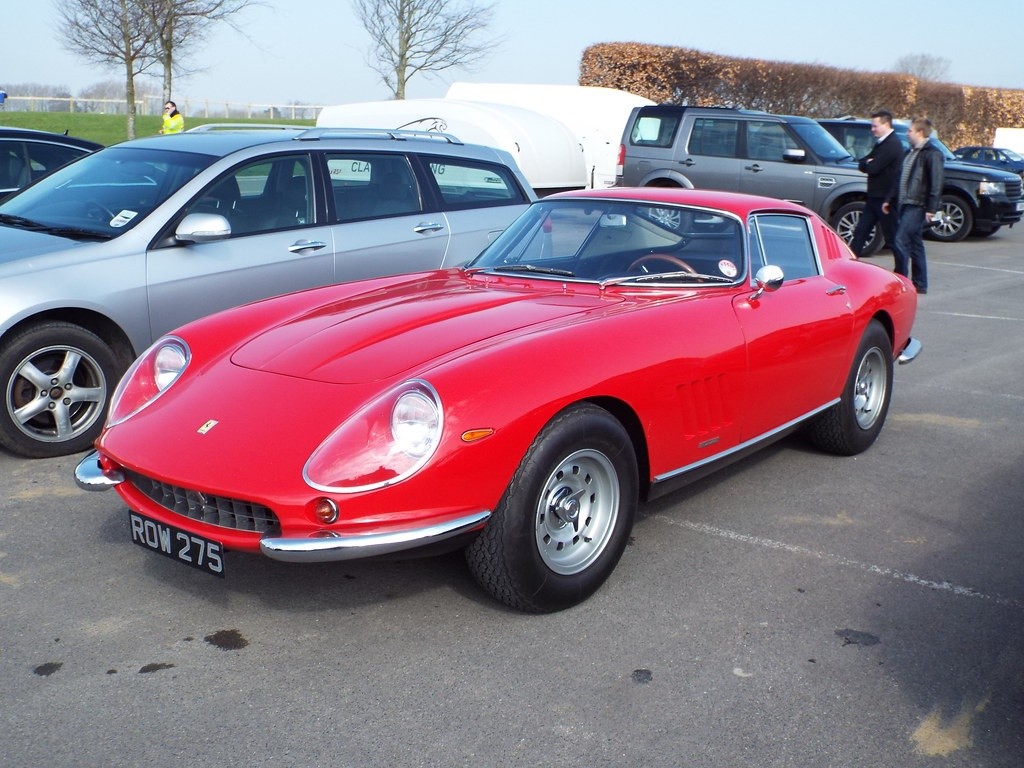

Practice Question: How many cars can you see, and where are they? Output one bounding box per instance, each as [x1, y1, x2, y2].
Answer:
[0, 126, 167, 206]
[950, 146, 1024, 173]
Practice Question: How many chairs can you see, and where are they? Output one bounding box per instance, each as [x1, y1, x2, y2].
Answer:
[270, 174, 308, 232]
[205, 174, 240, 206]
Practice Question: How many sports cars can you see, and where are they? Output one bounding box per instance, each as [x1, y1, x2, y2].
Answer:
[73, 187, 922, 614]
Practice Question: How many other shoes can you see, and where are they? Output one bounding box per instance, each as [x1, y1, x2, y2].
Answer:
[916, 290, 927, 294]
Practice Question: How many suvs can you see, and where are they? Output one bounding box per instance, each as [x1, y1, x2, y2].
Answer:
[0, 122, 552, 459]
[751, 113, 1024, 243]
[615, 105, 886, 257]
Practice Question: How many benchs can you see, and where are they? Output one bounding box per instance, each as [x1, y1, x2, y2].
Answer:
[633, 249, 808, 280]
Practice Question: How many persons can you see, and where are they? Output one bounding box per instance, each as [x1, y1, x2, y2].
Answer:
[0, 87, 7, 111]
[852, 111, 904, 273]
[881, 116, 944, 294]
[159, 101, 184, 135]
